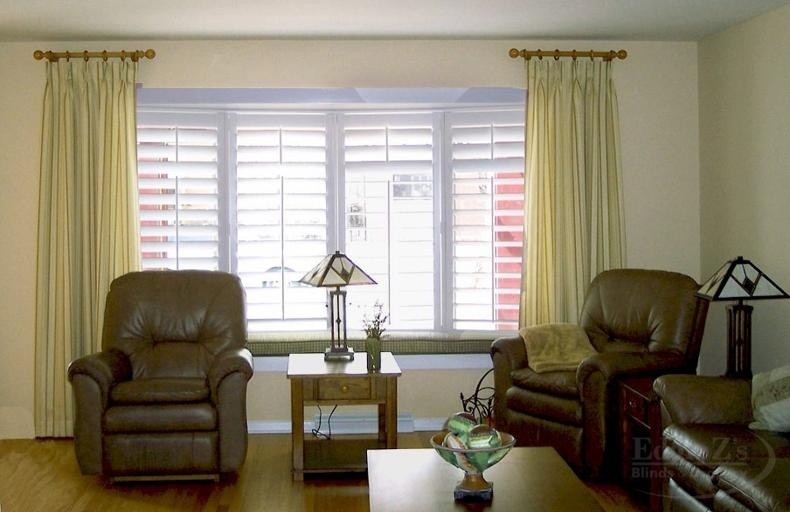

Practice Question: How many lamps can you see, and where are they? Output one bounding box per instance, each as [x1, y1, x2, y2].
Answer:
[695, 254, 790, 375]
[301, 251, 377, 361]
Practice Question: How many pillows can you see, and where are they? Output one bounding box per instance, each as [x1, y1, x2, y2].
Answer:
[748, 365, 790, 432]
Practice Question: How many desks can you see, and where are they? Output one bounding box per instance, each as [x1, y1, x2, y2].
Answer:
[286, 350, 400, 478]
[615, 375, 662, 512]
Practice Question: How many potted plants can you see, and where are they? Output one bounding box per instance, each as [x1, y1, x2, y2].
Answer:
[355, 304, 387, 373]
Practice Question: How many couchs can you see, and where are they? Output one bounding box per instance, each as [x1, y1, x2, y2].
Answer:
[650, 364, 790, 512]
[487, 264, 712, 474]
[67, 269, 256, 487]
